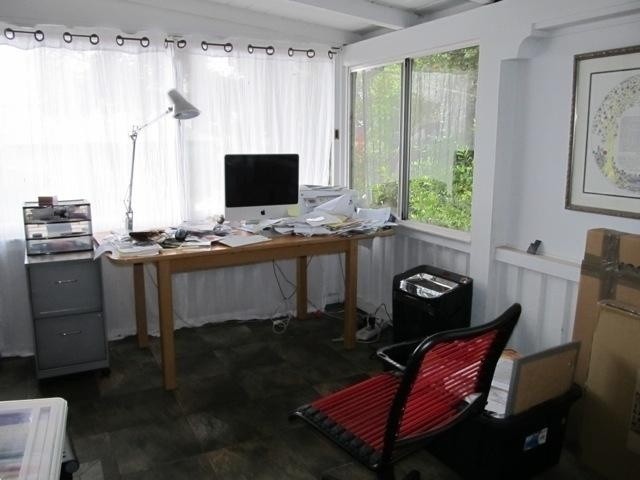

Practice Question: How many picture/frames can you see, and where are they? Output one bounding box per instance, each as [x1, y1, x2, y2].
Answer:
[563, 44, 639, 221]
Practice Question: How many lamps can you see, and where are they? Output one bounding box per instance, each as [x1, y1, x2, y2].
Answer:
[122, 88, 202, 230]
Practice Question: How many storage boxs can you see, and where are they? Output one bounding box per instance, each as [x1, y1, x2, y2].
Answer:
[573, 300, 639, 480]
[571, 228, 640, 394]
[0, 395, 69, 480]
[373, 338, 585, 479]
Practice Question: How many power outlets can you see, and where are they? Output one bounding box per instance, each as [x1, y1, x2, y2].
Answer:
[356, 325, 381, 340]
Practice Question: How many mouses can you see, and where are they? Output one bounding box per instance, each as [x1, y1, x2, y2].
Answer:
[175, 229, 186, 242]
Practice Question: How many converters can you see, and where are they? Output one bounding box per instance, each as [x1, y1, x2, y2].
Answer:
[368, 317, 376, 329]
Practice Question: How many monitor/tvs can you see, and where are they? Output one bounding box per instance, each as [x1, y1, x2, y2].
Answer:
[223, 154, 299, 230]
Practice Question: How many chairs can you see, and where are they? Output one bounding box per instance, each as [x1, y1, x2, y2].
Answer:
[287, 301, 523, 480]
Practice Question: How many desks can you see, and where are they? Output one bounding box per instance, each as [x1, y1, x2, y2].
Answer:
[92, 228, 396, 391]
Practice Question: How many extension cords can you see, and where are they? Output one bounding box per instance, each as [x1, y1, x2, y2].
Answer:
[356, 314, 389, 340]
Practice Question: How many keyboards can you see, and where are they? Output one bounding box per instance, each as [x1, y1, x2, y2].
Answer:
[218, 234, 272, 247]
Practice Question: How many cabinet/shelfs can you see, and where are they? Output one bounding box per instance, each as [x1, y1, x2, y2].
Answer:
[21, 197, 96, 258]
[22, 249, 111, 381]
